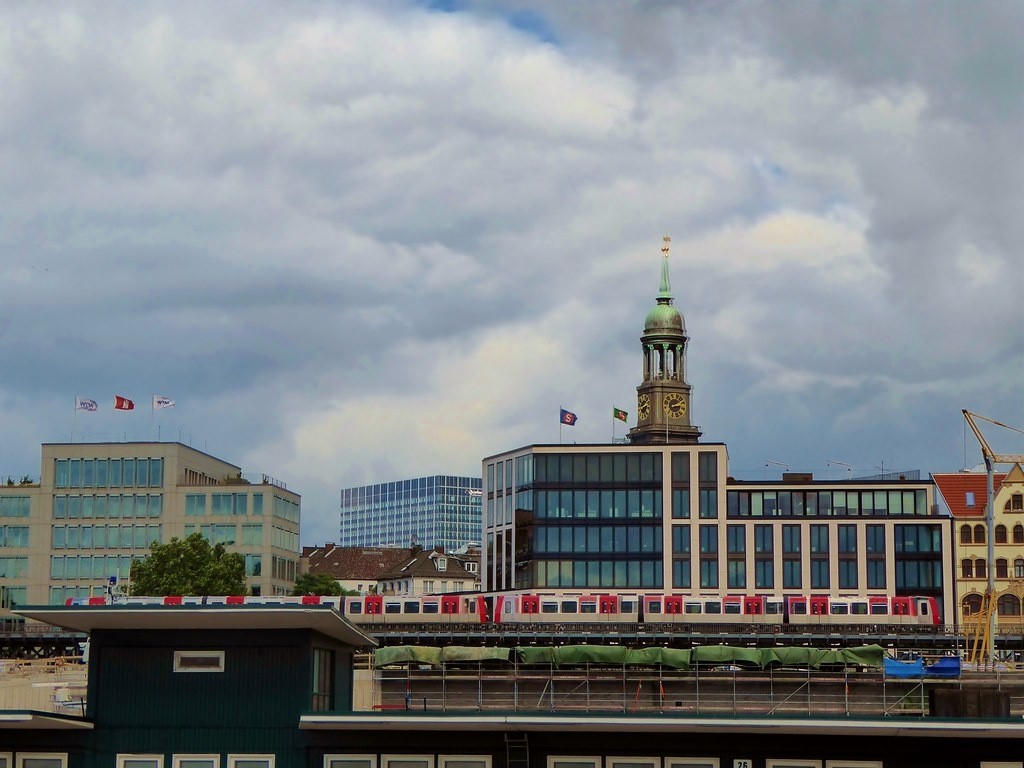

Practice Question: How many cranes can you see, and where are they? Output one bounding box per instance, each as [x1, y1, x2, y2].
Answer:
[962, 410, 1024, 670]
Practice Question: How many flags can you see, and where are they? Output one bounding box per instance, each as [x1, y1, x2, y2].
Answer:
[76, 396, 97, 413]
[153, 395, 175, 409]
[614, 408, 628, 424]
[560, 409, 577, 426]
[114, 396, 135, 411]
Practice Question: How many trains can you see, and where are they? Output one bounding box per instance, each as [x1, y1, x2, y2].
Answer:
[60, 592, 940, 637]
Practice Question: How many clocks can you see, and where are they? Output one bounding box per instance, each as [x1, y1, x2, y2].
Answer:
[637, 394, 651, 420]
[663, 393, 687, 419]
